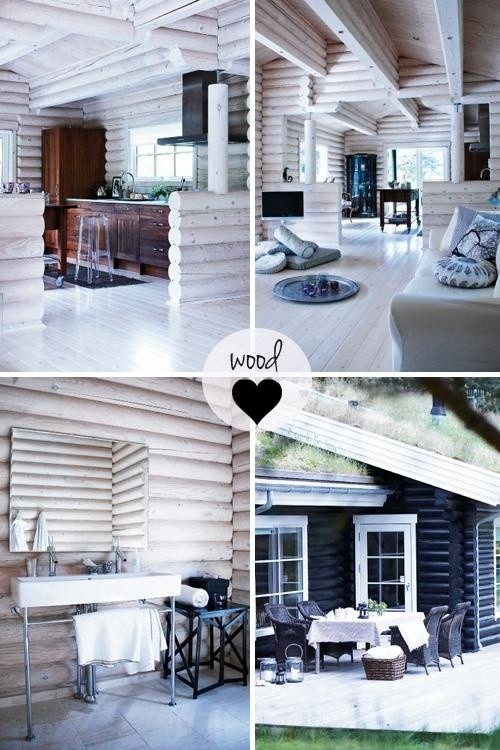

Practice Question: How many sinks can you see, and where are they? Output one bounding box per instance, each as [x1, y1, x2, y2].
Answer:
[10, 572, 181, 609]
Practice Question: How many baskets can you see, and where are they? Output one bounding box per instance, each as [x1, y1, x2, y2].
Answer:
[361, 655, 407, 680]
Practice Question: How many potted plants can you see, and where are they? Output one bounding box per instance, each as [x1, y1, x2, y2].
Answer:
[367, 599, 387, 616]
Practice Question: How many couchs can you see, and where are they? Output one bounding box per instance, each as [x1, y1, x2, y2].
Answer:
[388, 226, 500, 371]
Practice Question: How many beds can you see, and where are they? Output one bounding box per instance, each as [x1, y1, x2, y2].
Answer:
[165, 599, 249, 698]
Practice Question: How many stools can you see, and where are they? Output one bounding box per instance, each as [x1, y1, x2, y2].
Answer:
[73, 215, 113, 283]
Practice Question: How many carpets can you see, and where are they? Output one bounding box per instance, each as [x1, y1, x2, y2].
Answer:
[45, 262, 149, 289]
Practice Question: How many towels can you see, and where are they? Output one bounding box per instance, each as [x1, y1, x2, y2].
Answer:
[178, 586, 208, 606]
[72, 605, 166, 675]
[10, 508, 29, 551]
[32, 504, 50, 551]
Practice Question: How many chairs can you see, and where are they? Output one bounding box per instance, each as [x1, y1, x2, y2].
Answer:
[264, 604, 325, 672]
[438, 601, 472, 668]
[389, 606, 448, 675]
[342, 191, 355, 224]
[297, 602, 354, 664]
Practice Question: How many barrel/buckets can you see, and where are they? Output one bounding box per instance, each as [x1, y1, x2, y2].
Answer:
[156, 68, 216, 147]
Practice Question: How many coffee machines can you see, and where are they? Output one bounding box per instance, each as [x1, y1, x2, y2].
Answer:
[112, 176, 123, 197]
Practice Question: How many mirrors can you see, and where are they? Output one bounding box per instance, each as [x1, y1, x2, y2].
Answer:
[10, 427, 149, 553]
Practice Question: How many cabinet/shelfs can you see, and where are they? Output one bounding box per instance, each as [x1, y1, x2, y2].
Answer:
[114, 200, 138, 270]
[138, 200, 171, 269]
[344, 154, 377, 218]
[183, 70, 217, 137]
[63, 196, 114, 267]
[44, 128, 105, 205]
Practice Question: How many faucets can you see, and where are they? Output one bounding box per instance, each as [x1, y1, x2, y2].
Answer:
[115, 548, 126, 572]
[479, 167, 491, 178]
[119, 170, 136, 194]
[48, 548, 58, 575]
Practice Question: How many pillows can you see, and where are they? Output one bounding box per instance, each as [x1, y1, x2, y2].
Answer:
[434, 206, 500, 288]
[256, 225, 340, 275]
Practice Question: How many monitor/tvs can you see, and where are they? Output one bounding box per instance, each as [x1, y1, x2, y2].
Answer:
[260, 191, 305, 220]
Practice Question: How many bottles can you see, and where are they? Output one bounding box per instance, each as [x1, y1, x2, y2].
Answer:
[356, 602, 369, 619]
[275, 668, 285, 683]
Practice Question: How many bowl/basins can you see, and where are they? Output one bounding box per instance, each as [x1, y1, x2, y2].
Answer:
[388, 180, 400, 188]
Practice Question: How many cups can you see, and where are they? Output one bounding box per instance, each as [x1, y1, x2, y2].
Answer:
[302, 272, 320, 296]
[393, 182, 399, 189]
[335, 609, 345, 616]
[214, 593, 225, 610]
[143, 193, 150, 199]
[345, 608, 355, 616]
[331, 281, 339, 289]
[24, 558, 38, 577]
[408, 182, 411, 189]
[325, 610, 334, 618]
[316, 272, 329, 296]
[16, 182, 30, 193]
[40, 192, 49, 204]
[134, 194, 142, 199]
[1, 182, 15, 193]
[400, 184, 405, 189]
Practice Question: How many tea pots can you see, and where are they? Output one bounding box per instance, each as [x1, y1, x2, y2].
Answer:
[96, 186, 106, 196]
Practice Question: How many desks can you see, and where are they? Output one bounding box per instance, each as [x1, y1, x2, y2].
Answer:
[379, 188, 422, 232]
